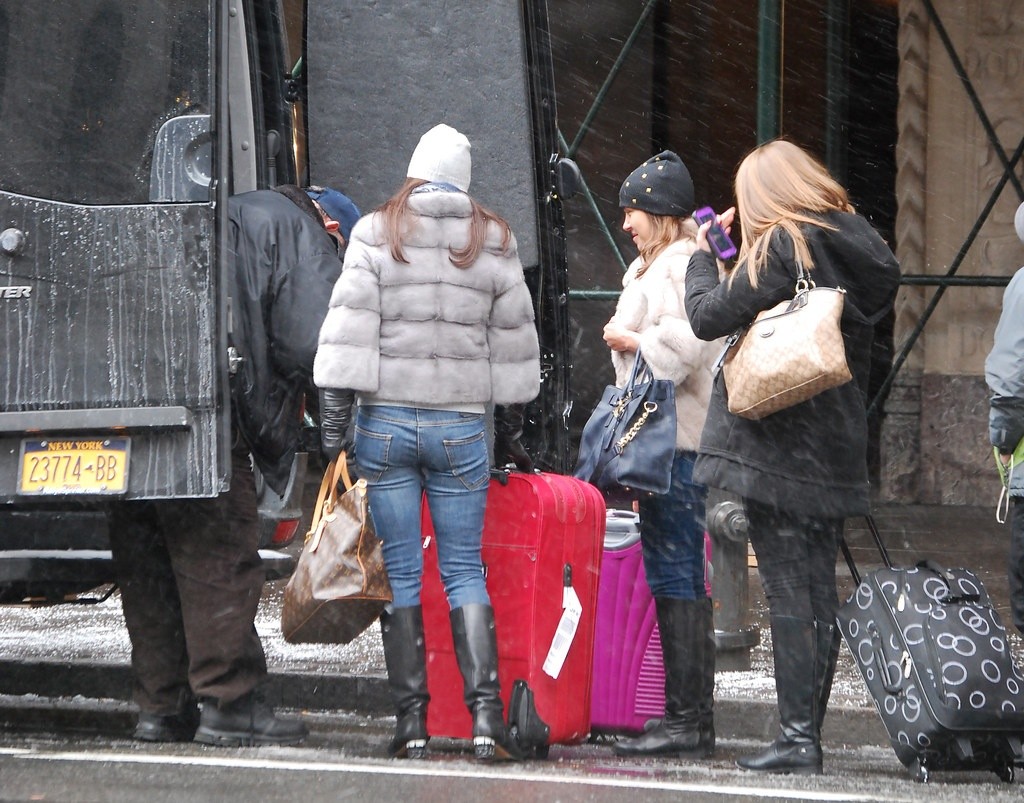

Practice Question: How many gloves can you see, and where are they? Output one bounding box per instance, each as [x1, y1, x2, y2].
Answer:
[493, 402, 535, 473]
[317, 387, 357, 466]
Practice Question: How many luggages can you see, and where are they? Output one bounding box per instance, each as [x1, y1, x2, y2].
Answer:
[417, 463, 608, 757]
[590, 506, 715, 741]
[834, 514, 1024, 785]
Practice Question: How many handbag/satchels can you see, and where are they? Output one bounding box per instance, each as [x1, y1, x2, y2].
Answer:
[570, 345, 678, 496]
[711, 223, 852, 422]
[281, 449, 393, 646]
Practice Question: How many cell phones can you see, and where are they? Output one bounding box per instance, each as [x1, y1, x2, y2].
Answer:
[693, 205, 737, 260]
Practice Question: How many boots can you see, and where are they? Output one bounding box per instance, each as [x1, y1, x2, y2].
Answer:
[378, 604, 431, 759]
[610, 595, 716, 759]
[735, 613, 842, 775]
[451, 604, 519, 764]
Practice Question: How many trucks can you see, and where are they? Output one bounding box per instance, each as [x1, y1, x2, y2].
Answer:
[0, 0, 572, 612]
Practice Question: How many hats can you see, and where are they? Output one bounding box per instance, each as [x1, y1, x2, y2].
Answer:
[406, 123, 472, 193]
[618, 150, 695, 218]
[306, 184, 361, 241]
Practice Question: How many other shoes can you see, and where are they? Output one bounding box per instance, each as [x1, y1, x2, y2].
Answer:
[193, 689, 310, 745]
[133, 709, 200, 743]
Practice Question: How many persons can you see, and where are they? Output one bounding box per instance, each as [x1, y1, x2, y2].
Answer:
[982, 205, 1023, 633]
[311, 127, 542, 763]
[682, 137, 902, 777]
[603, 150, 724, 760]
[103, 184, 360, 749]
